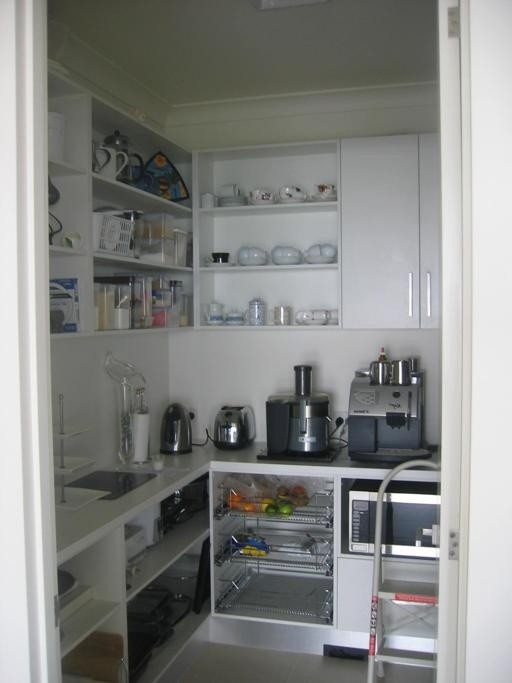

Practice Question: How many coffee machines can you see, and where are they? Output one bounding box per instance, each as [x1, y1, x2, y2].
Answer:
[348, 369, 431, 462]
[267, 391, 331, 458]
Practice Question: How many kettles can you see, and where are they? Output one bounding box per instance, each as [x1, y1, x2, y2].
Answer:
[158, 403, 194, 454]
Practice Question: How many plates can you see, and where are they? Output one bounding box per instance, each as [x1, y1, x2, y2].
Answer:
[311, 195, 337, 201]
[205, 261, 234, 268]
[276, 196, 306, 202]
[217, 195, 248, 207]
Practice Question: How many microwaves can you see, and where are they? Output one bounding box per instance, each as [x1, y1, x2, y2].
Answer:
[346, 479, 439, 559]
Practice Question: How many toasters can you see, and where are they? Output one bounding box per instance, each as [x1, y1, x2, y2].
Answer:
[213, 404, 256, 449]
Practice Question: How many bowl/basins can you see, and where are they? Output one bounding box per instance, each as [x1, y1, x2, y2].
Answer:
[238, 258, 266, 265]
[273, 255, 300, 265]
[306, 256, 333, 262]
[277, 184, 307, 199]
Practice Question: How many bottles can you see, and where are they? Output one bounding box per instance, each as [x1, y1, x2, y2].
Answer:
[114, 375, 152, 468]
[368, 347, 418, 386]
[170, 278, 191, 325]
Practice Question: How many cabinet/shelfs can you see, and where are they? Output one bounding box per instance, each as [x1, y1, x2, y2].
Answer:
[197, 140, 339, 332]
[210, 459, 334, 631]
[338, 131, 440, 328]
[49, 71, 192, 339]
[53, 456, 207, 681]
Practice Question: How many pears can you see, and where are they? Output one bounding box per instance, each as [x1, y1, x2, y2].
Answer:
[260, 498, 274, 512]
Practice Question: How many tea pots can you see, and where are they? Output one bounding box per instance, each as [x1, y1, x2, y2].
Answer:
[204, 302, 228, 325]
[92, 142, 128, 181]
[225, 306, 244, 324]
[104, 128, 145, 185]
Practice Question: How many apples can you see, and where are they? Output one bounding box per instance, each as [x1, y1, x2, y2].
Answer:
[244, 502, 256, 512]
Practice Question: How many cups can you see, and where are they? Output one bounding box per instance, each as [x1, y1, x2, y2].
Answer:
[244, 297, 264, 326]
[268, 304, 291, 326]
[294, 365, 313, 395]
[236, 247, 266, 257]
[151, 455, 165, 471]
[202, 191, 216, 208]
[302, 243, 338, 258]
[212, 252, 228, 262]
[249, 188, 273, 204]
[294, 309, 338, 325]
[315, 183, 334, 195]
[272, 244, 301, 256]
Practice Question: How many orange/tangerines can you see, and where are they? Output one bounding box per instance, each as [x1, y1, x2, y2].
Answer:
[279, 502, 292, 517]
[266, 505, 276, 515]
